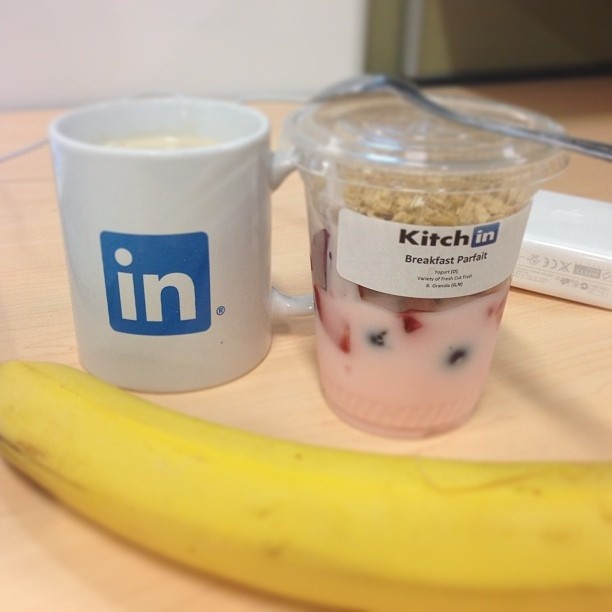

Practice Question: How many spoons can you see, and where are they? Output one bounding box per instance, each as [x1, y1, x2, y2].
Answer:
[311, 72, 612, 164]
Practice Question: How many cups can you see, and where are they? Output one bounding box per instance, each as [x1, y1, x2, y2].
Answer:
[282, 88, 575, 440]
[50, 98, 316, 393]
